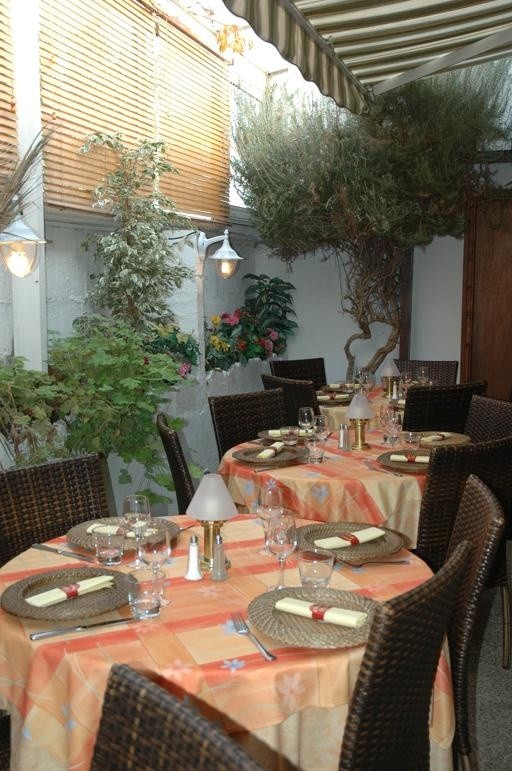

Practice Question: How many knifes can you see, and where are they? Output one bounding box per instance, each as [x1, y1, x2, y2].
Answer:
[31, 541, 88, 563]
[254, 463, 298, 472]
[29, 618, 135, 638]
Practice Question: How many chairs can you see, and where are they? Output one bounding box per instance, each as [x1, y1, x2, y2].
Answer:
[412, 435, 512, 670]
[338, 539, 474, 769]
[156, 412, 197, 513]
[393, 359, 459, 386]
[269, 357, 327, 391]
[89, 662, 266, 770]
[261, 372, 321, 427]
[462, 394, 512, 444]
[402, 380, 488, 434]
[0, 450, 119, 566]
[440, 474, 505, 770]
[209, 387, 289, 464]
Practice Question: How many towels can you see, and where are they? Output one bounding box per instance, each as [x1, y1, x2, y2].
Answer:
[316, 393, 350, 401]
[421, 433, 451, 443]
[26, 576, 115, 609]
[390, 455, 430, 463]
[257, 442, 284, 459]
[398, 400, 405, 405]
[268, 429, 314, 437]
[274, 598, 368, 628]
[87, 523, 157, 540]
[329, 384, 367, 389]
[314, 527, 385, 550]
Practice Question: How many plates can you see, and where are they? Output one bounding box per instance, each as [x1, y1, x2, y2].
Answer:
[316, 393, 353, 404]
[67, 516, 178, 550]
[1, 567, 140, 619]
[403, 431, 470, 444]
[258, 430, 315, 441]
[290, 521, 410, 558]
[377, 449, 436, 473]
[233, 445, 307, 461]
[389, 398, 407, 410]
[321, 383, 351, 392]
[247, 586, 378, 648]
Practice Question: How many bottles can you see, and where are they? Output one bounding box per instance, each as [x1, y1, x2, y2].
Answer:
[391, 381, 398, 399]
[209, 534, 230, 581]
[343, 425, 352, 452]
[184, 535, 203, 581]
[339, 424, 344, 449]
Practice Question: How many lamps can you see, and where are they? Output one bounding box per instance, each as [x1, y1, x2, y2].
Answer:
[1, 193, 47, 279]
[186, 473, 239, 575]
[209, 230, 244, 278]
[379, 355, 400, 400]
[345, 394, 374, 452]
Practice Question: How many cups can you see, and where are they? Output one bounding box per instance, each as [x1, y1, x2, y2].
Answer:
[128, 496, 151, 569]
[314, 414, 330, 448]
[257, 492, 280, 555]
[92, 526, 124, 565]
[304, 438, 323, 463]
[266, 508, 295, 592]
[388, 413, 400, 451]
[343, 379, 356, 393]
[300, 408, 314, 447]
[379, 404, 394, 446]
[406, 433, 422, 460]
[297, 549, 336, 584]
[361, 370, 376, 393]
[281, 426, 299, 445]
[130, 570, 162, 621]
[417, 366, 429, 385]
[400, 370, 411, 384]
[140, 524, 172, 604]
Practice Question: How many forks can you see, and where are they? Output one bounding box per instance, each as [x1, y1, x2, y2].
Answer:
[338, 555, 414, 568]
[229, 610, 277, 659]
